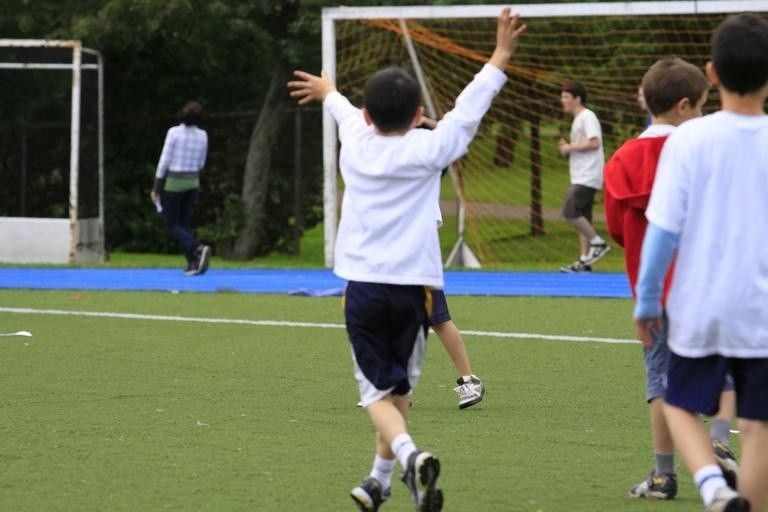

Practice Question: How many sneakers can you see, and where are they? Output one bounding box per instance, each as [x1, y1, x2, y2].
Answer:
[583, 241, 610, 266]
[711, 437, 737, 490]
[559, 259, 591, 274]
[350, 477, 385, 512]
[627, 470, 676, 500]
[453, 375, 485, 409]
[400, 451, 442, 512]
[704, 487, 749, 512]
[193, 244, 210, 275]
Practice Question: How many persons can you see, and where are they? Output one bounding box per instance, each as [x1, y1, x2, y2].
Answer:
[413, 116, 486, 410]
[555, 81, 613, 273]
[150, 100, 214, 276]
[286, 8, 528, 512]
[637, 85, 657, 126]
[634, 8, 768, 511]
[602, 54, 739, 499]
